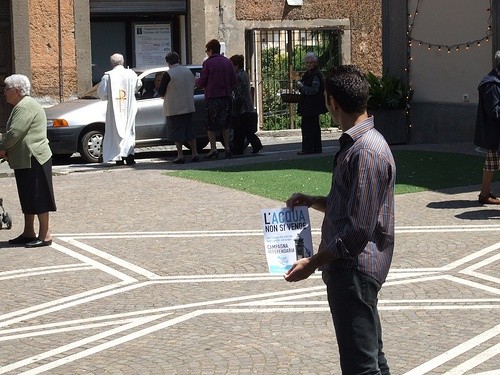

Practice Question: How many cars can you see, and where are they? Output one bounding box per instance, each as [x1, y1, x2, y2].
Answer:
[44, 63, 259, 165]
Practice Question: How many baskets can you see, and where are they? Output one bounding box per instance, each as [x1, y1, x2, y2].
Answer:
[281, 82, 300, 103]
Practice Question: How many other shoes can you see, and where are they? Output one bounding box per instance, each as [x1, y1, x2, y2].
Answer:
[25, 238, 52, 247]
[296, 150, 309, 155]
[479, 193, 500, 204]
[251, 145, 263, 154]
[8, 235, 36, 244]
[128, 161, 135, 165]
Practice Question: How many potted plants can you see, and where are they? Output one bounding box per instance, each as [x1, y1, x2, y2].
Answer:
[364, 68, 411, 144]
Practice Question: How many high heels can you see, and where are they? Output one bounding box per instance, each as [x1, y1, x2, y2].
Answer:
[203, 150, 219, 160]
[191, 155, 199, 162]
[225, 149, 231, 159]
[172, 158, 185, 164]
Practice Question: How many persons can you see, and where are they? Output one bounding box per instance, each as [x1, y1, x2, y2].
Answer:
[193, 39, 241, 159]
[0, 74, 57, 248]
[97, 54, 142, 165]
[296, 52, 329, 155]
[230, 55, 264, 155]
[284, 67, 396, 375]
[158, 51, 199, 163]
[474, 52, 500, 204]
[140, 72, 164, 100]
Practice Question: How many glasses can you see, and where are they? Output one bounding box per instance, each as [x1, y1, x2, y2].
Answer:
[3, 87, 14, 93]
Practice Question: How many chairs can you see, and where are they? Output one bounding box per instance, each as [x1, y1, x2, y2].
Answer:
[142, 78, 155, 99]
